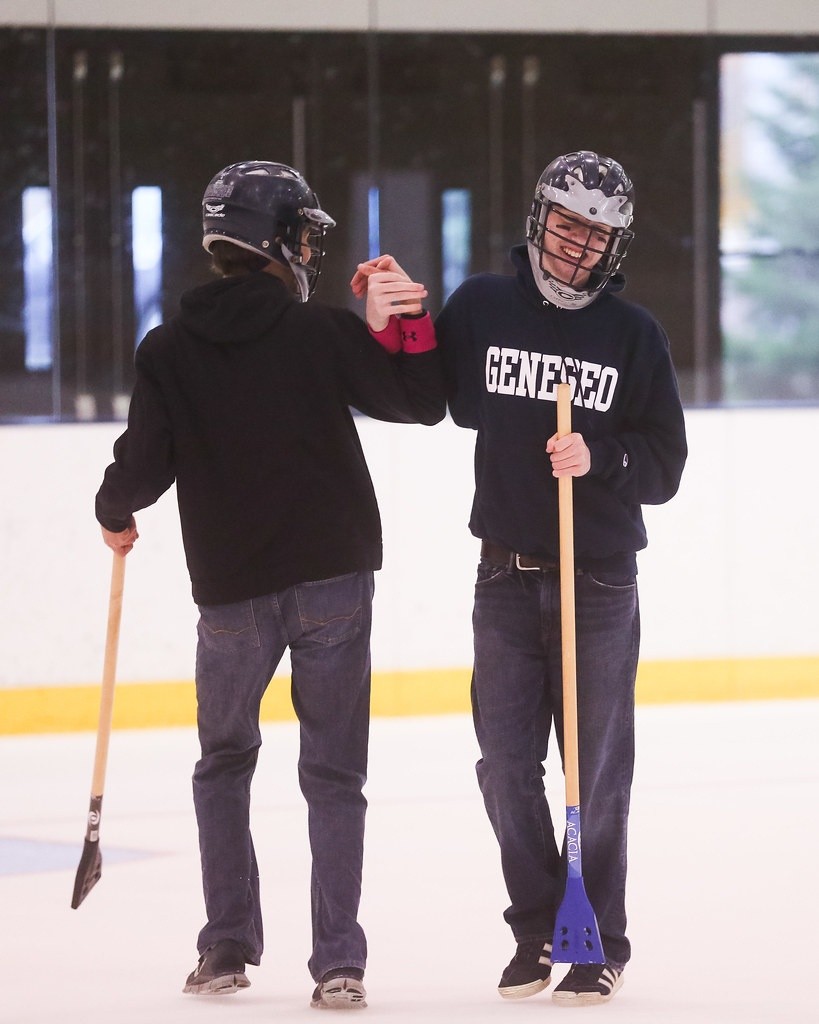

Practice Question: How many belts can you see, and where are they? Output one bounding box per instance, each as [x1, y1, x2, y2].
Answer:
[481, 542, 557, 571]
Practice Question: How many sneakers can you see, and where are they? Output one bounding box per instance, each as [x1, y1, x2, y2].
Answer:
[498, 942, 564, 1000]
[309, 968, 367, 1010]
[184, 940, 250, 995]
[551, 959, 623, 1007]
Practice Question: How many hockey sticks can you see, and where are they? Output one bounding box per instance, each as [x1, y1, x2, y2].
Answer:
[552, 384, 606, 967]
[76, 551, 128, 905]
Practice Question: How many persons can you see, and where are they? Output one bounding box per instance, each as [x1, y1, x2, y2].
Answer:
[364, 151, 690, 1003]
[93, 159, 444, 1011]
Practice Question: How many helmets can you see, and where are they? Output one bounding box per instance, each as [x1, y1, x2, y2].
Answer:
[525, 151, 635, 311]
[201, 160, 335, 303]
